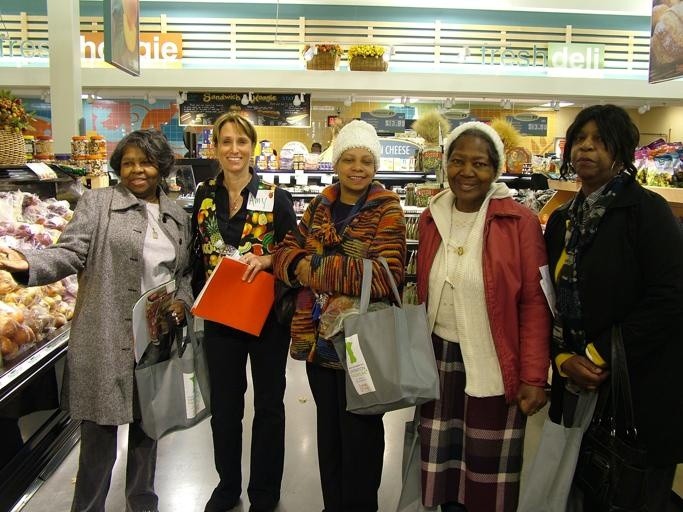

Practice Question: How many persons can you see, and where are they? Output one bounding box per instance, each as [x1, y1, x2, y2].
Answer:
[416, 122, 550, 511]
[190, 112, 298, 512]
[0, 126, 191, 512]
[517, 103, 683, 512]
[273, 119, 407, 512]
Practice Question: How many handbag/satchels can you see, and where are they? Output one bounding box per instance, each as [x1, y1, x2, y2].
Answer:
[518, 388, 599, 512]
[345, 256, 441, 413]
[396, 406, 439, 512]
[568, 328, 648, 512]
[136, 301, 210, 441]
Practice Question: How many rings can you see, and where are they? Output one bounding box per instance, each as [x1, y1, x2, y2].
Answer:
[171, 311, 177, 317]
[249, 264, 256, 267]
[176, 323, 180, 325]
[176, 320, 180, 323]
[175, 317, 179, 321]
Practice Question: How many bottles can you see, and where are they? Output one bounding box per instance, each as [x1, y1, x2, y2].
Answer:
[293, 161, 298, 170]
[299, 154, 304, 161]
[293, 154, 299, 161]
[298, 161, 304, 169]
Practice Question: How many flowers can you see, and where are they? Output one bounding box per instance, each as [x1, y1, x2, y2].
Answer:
[0, 88, 37, 132]
[302, 42, 343, 57]
[346, 44, 386, 61]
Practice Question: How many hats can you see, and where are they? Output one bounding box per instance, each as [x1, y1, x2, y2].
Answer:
[441, 121, 505, 183]
[332, 120, 383, 172]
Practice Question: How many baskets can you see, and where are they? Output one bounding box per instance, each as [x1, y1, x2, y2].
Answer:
[349, 56, 388, 71]
[0, 129, 26, 166]
[305, 51, 340, 71]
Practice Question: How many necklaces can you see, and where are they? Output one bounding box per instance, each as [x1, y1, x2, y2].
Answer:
[224, 180, 244, 215]
[144, 214, 168, 243]
